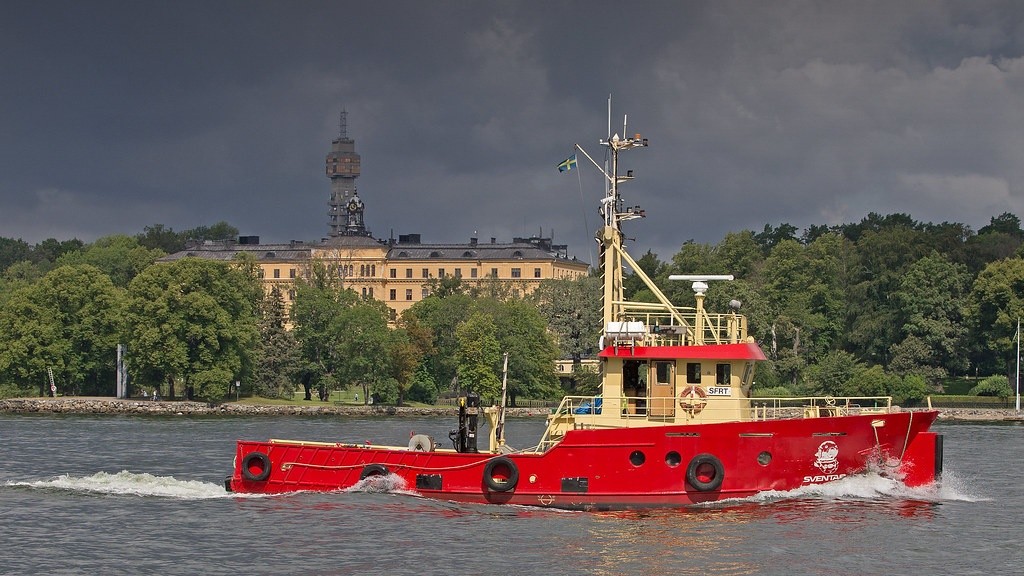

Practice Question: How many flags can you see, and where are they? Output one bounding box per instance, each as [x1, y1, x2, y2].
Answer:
[557, 155, 576, 173]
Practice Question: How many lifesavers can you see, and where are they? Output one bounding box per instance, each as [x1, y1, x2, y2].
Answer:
[359, 465, 390, 480]
[687, 454, 725, 492]
[680, 386, 707, 414]
[483, 457, 519, 493]
[242, 452, 271, 482]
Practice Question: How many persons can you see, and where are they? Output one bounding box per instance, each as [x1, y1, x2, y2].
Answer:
[355, 393, 358, 401]
[140, 388, 148, 396]
[153, 389, 157, 401]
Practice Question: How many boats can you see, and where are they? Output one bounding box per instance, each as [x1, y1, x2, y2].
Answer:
[225, 96, 943, 512]
